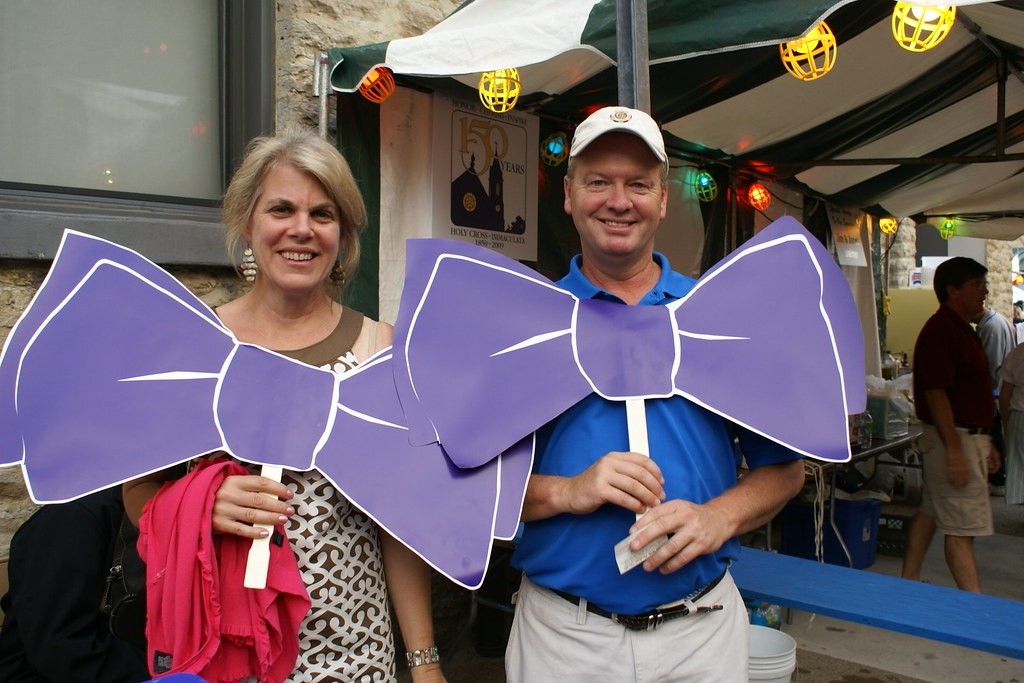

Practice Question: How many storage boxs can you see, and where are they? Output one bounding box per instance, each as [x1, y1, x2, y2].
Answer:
[876, 499, 923, 558]
[782, 497, 878, 569]
[867, 393, 911, 440]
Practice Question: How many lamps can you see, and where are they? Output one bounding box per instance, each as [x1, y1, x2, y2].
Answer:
[357, 67, 396, 103]
[941, 219, 955, 240]
[891, 0, 956, 52]
[879, 217, 899, 235]
[540, 131, 570, 167]
[694, 171, 717, 202]
[780, 20, 838, 82]
[477, 68, 522, 114]
[749, 183, 771, 211]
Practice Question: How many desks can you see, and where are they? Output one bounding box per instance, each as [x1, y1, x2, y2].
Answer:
[739, 423, 925, 554]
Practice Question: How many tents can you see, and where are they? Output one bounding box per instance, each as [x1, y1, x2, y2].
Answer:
[323, 0, 1023, 378]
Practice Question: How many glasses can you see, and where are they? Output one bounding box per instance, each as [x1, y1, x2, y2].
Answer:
[953, 280, 990, 288]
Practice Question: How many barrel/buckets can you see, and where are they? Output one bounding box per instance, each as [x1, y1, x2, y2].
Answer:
[749, 625, 797, 683]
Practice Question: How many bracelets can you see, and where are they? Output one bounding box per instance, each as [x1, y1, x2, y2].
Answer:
[406, 646, 440, 668]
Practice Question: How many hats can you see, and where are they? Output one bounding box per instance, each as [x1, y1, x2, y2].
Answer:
[568, 105, 670, 179]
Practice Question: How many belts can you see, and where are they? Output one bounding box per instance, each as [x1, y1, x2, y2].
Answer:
[923, 420, 994, 435]
[549, 566, 728, 632]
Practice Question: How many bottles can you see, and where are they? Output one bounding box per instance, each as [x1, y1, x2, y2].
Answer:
[882, 350, 896, 381]
[902, 353, 910, 367]
[855, 410, 873, 449]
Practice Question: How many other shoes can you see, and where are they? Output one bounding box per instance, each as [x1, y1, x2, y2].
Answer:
[990, 484, 1005, 496]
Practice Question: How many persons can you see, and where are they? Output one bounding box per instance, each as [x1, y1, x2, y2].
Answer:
[123, 130, 448, 683]
[503, 106, 808, 683]
[1014, 300, 1024, 327]
[902, 257, 1000, 594]
[970, 306, 1016, 495]
[1001, 343, 1024, 505]
